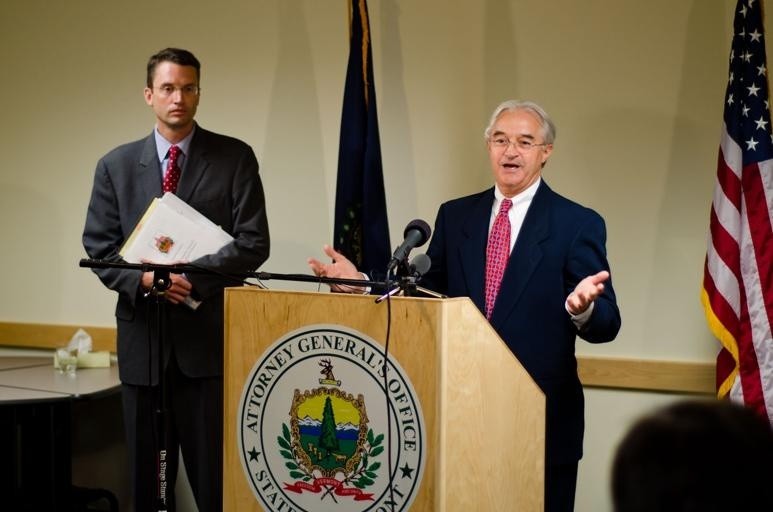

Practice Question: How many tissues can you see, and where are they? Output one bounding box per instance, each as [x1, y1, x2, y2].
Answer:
[54, 329, 110, 369]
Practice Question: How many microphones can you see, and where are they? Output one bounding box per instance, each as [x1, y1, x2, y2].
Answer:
[409, 253, 431, 282]
[390, 219, 431, 269]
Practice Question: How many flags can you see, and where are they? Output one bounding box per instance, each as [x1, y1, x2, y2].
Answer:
[331, 0, 393, 293]
[701, 0, 773, 422]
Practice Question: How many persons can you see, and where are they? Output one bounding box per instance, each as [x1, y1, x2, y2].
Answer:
[611, 401, 773, 512]
[310, 100, 621, 512]
[82, 49, 269, 512]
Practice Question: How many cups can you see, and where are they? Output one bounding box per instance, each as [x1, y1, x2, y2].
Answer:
[55, 343, 80, 375]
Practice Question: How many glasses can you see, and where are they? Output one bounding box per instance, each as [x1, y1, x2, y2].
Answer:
[484, 135, 547, 152]
[148, 83, 201, 97]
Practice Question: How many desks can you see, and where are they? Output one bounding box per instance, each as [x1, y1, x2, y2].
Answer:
[0, 344, 122, 508]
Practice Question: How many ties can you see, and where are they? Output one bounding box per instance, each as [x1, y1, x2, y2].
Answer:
[481, 197, 513, 322]
[157, 145, 183, 197]
